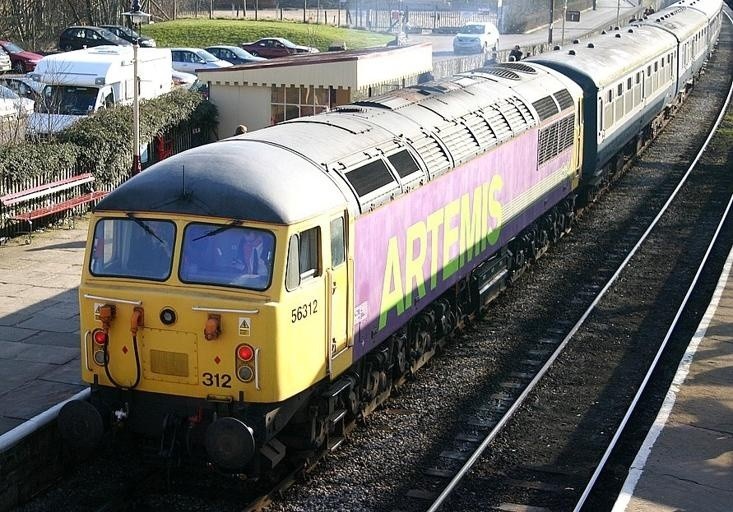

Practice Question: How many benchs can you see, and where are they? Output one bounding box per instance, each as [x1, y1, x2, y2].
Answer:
[1, 171, 109, 245]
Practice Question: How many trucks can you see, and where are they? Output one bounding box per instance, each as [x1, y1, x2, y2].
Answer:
[24, 44, 172, 145]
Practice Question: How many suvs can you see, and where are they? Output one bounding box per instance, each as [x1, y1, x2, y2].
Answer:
[98, 25, 156, 48]
[203, 46, 267, 66]
[0, 45, 11, 75]
[59, 26, 133, 53]
[451, 22, 499, 54]
[477, 4, 489, 16]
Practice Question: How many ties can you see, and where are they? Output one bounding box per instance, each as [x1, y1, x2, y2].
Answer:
[252, 248, 258, 275]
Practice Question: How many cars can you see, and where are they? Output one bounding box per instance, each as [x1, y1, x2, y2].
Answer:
[0, 84, 35, 121]
[241, 38, 319, 59]
[0, 41, 43, 74]
[0, 75, 50, 101]
[169, 48, 233, 73]
[170, 68, 198, 87]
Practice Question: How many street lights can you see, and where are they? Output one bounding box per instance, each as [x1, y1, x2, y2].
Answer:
[119, 1, 151, 176]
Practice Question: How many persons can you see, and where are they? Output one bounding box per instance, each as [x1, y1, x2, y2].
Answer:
[481, 5, 655, 65]
[236, 228, 271, 273]
[234, 125, 247, 138]
[127, 223, 172, 275]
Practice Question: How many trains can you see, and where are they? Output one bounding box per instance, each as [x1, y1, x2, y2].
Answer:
[57, 0, 722, 484]
